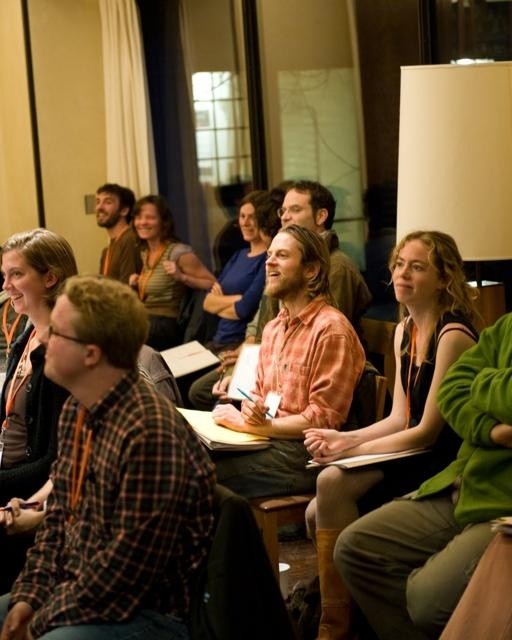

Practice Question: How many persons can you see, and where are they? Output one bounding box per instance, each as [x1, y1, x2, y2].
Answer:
[1, 274, 218, 640]
[1, 473, 55, 537]
[333, 309, 511, 640]
[1, 183, 373, 412]
[1, 228, 78, 507]
[204, 222, 368, 500]
[301, 228, 479, 640]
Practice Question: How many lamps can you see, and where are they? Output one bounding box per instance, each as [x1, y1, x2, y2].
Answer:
[394, 59, 510, 333]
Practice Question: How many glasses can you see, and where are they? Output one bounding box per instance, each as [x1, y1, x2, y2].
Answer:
[45, 324, 93, 346]
[275, 204, 312, 219]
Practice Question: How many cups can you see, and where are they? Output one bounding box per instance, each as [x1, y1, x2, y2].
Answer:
[278, 563, 291, 603]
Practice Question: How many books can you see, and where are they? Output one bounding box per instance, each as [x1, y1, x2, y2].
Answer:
[304, 443, 432, 470]
[175, 407, 272, 452]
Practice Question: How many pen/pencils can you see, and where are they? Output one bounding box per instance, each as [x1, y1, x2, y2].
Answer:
[0, 501, 40, 511]
[219, 370, 227, 392]
[237, 388, 275, 418]
